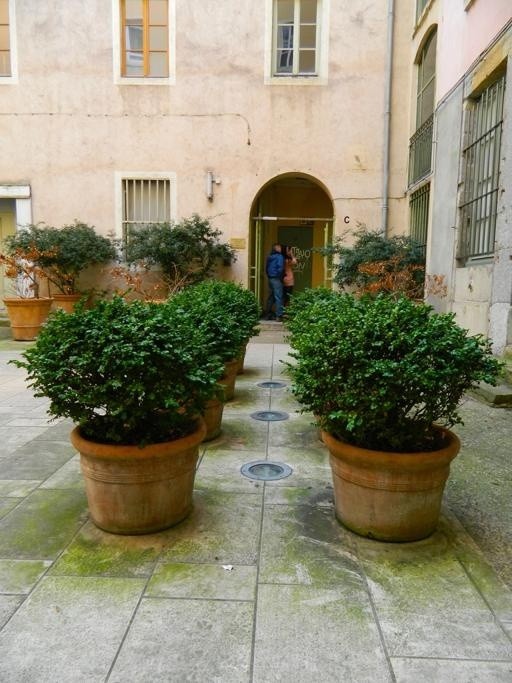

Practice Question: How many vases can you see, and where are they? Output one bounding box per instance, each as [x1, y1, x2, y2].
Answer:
[0, 253, 54, 341]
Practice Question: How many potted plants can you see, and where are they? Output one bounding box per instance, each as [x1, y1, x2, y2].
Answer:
[118, 214, 238, 304]
[283, 280, 394, 443]
[4, 292, 207, 537]
[1, 219, 120, 313]
[155, 287, 230, 443]
[205, 277, 264, 374]
[278, 291, 508, 545]
[177, 280, 242, 404]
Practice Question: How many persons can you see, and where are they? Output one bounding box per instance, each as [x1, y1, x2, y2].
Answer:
[265, 243, 285, 322]
[282, 244, 297, 306]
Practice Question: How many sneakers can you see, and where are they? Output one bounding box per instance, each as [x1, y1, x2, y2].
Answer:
[266, 314, 276, 320]
[275, 316, 286, 321]
